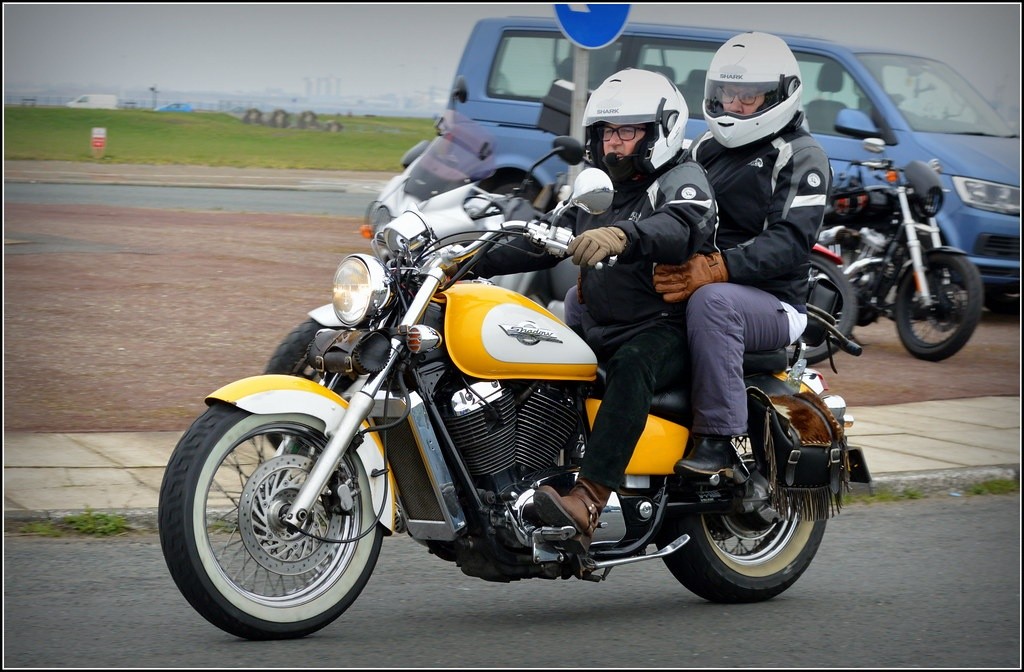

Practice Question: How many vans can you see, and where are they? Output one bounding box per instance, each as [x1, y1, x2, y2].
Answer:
[445, 15, 1021, 307]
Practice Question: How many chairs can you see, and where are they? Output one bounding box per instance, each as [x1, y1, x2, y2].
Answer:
[642, 63, 676, 84]
[803, 63, 848, 136]
[686, 69, 708, 115]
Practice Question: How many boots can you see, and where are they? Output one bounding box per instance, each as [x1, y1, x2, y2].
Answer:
[533, 478, 611, 553]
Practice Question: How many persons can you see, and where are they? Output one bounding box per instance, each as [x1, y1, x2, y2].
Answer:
[447, 66, 724, 553]
[563, 31, 835, 485]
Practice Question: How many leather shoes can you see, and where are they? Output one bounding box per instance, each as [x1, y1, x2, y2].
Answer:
[673, 437, 733, 480]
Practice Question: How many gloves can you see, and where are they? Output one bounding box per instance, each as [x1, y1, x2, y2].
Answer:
[653, 252, 730, 302]
[567, 226, 627, 268]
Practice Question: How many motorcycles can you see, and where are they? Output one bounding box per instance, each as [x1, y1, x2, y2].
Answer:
[359, 72, 987, 368]
[157, 168, 872, 642]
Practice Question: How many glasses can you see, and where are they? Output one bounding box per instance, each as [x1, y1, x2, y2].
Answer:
[598, 125, 646, 142]
[720, 89, 774, 106]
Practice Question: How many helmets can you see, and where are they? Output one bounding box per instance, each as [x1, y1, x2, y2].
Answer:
[703, 31, 803, 149]
[583, 68, 689, 186]
